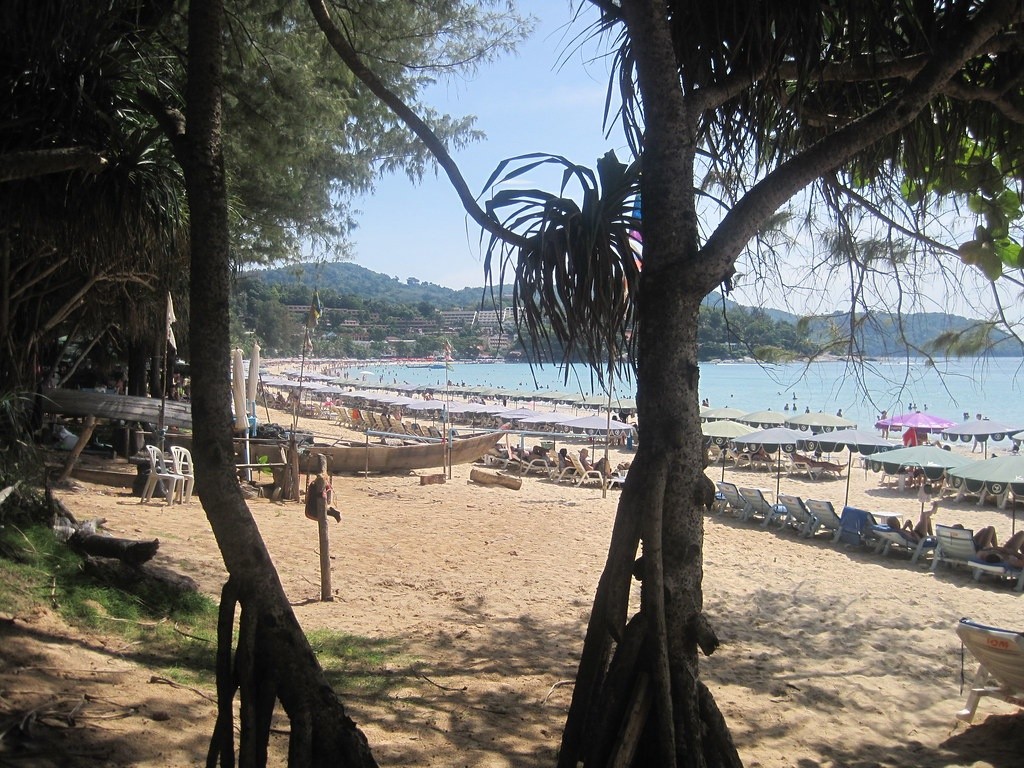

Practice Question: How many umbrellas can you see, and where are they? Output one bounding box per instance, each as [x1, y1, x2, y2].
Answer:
[700, 406, 1024, 536]
[230, 359, 637, 464]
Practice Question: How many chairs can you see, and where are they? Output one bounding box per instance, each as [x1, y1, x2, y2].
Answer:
[137, 383, 1024, 719]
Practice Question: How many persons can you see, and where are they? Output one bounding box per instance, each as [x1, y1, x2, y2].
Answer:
[735, 393, 1024, 567]
[259, 361, 637, 479]
